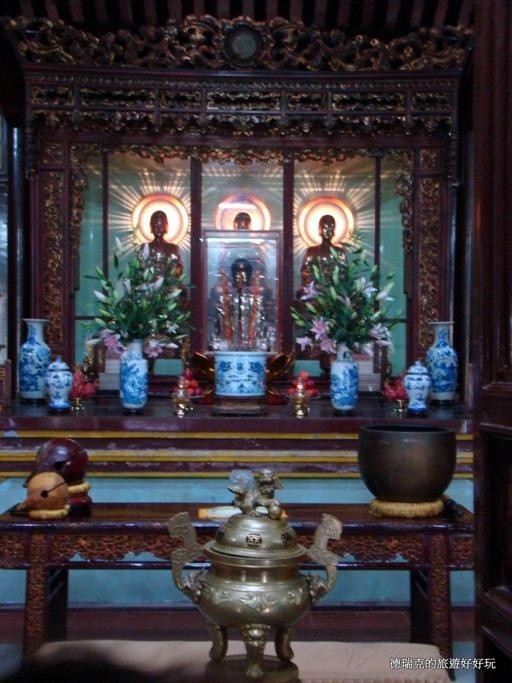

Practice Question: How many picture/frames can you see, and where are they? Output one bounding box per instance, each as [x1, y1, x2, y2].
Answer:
[197, 228, 286, 354]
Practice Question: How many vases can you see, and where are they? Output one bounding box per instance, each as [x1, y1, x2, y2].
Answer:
[119, 339, 152, 413]
[328, 343, 361, 413]
[405, 318, 457, 414]
[17, 316, 74, 411]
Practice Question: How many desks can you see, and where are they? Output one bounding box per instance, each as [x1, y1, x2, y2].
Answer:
[0, 500, 480, 681]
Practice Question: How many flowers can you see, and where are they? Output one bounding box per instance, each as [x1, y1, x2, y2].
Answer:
[289, 240, 400, 355]
[78, 233, 190, 361]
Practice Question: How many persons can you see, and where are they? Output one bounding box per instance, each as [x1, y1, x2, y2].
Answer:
[209, 211, 273, 300]
[210, 256, 273, 353]
[137, 208, 189, 300]
[296, 213, 346, 299]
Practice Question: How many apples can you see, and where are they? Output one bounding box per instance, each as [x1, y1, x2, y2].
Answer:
[70, 370, 97, 396]
[287, 371, 319, 397]
[184, 368, 203, 396]
